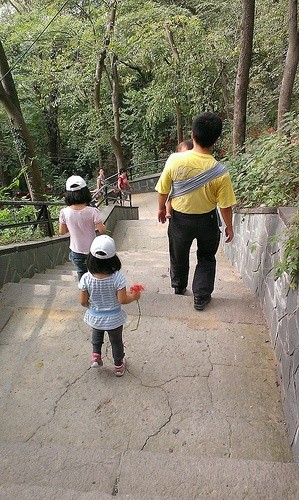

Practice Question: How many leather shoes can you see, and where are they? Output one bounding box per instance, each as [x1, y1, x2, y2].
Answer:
[194, 295, 212, 310]
[176, 287, 186, 295]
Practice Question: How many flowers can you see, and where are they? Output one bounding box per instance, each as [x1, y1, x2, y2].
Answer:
[129, 284, 145, 332]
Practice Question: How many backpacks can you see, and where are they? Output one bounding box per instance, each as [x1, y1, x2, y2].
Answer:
[113, 177, 123, 193]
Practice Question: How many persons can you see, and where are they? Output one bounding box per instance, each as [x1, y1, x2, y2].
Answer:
[155, 117, 235, 310]
[77, 235, 141, 376]
[115, 168, 129, 202]
[55, 174, 105, 281]
[96, 167, 108, 201]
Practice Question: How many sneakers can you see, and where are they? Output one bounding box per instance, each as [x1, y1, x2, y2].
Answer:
[114, 358, 126, 376]
[91, 354, 103, 367]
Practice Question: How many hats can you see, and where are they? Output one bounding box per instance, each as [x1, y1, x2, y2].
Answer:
[90, 234, 116, 259]
[66, 176, 87, 191]
